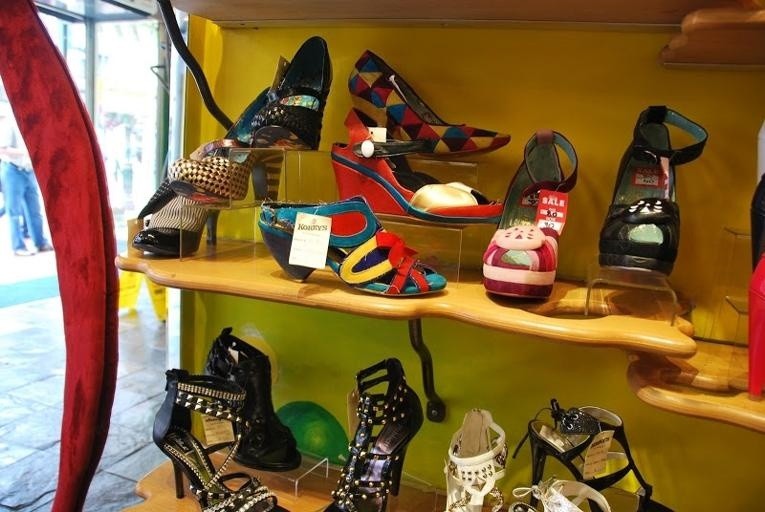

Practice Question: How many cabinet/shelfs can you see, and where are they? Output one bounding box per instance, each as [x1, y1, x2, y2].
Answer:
[112, 0, 764, 511]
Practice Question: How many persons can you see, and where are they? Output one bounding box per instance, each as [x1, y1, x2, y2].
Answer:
[0, 118, 54, 257]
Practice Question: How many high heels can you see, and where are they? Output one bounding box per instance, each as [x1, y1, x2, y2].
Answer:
[152, 368, 291, 512]
[167, 86, 283, 200]
[133, 179, 220, 257]
[331, 107, 504, 222]
[347, 49, 510, 156]
[258, 195, 447, 296]
[324, 358, 423, 510]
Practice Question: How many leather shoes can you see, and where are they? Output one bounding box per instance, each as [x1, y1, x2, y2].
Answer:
[600, 107, 708, 275]
[250, 36, 333, 150]
[483, 130, 577, 300]
[206, 327, 301, 470]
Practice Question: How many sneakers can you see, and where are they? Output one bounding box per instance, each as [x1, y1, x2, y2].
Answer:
[15, 249, 34, 256]
[39, 244, 53, 252]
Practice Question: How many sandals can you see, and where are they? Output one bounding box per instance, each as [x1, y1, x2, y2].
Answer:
[445, 398, 652, 512]
